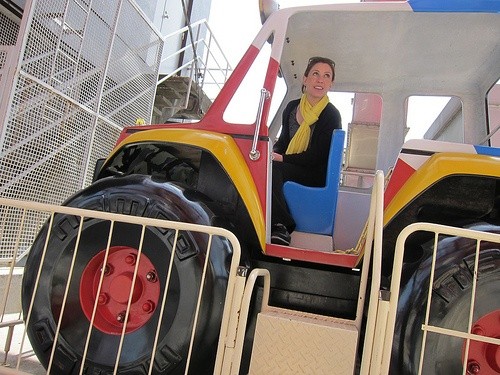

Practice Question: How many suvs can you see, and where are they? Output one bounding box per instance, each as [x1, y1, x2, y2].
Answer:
[20, 0, 500, 375]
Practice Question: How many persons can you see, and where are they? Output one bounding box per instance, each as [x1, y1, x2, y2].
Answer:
[271, 56, 342, 245]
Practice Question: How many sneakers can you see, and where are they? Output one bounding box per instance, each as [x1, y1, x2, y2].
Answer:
[271, 222, 292, 245]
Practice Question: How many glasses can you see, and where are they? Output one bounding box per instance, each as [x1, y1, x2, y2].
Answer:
[308, 57, 335, 67]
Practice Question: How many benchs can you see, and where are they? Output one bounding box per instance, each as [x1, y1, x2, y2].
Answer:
[283, 128, 346, 236]
